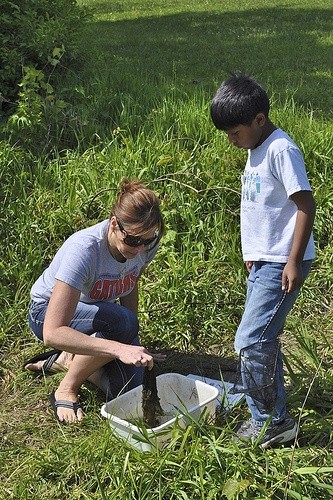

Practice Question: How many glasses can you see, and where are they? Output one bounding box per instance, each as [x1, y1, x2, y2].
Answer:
[111, 211, 158, 247]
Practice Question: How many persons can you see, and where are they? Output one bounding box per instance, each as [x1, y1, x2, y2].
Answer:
[209, 78, 317, 450]
[21, 183, 164, 425]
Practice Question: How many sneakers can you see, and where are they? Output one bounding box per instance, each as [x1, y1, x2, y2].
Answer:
[227, 411, 300, 452]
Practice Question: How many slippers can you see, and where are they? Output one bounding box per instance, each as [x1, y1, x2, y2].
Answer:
[22, 348, 64, 375]
[50, 390, 87, 425]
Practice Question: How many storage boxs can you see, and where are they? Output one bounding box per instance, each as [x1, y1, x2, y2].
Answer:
[100, 373, 219, 453]
